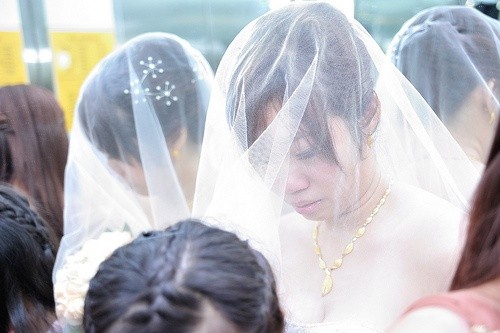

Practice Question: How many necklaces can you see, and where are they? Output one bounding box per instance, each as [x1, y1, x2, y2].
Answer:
[313, 172, 394, 296]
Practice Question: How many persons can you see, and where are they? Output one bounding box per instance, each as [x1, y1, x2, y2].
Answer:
[0, 83, 70, 241]
[384, 147, 500, 333]
[192, 0, 480, 333]
[82, 219, 285, 332]
[51, 33, 214, 332]
[0, 187, 63, 333]
[384, 5, 500, 176]
